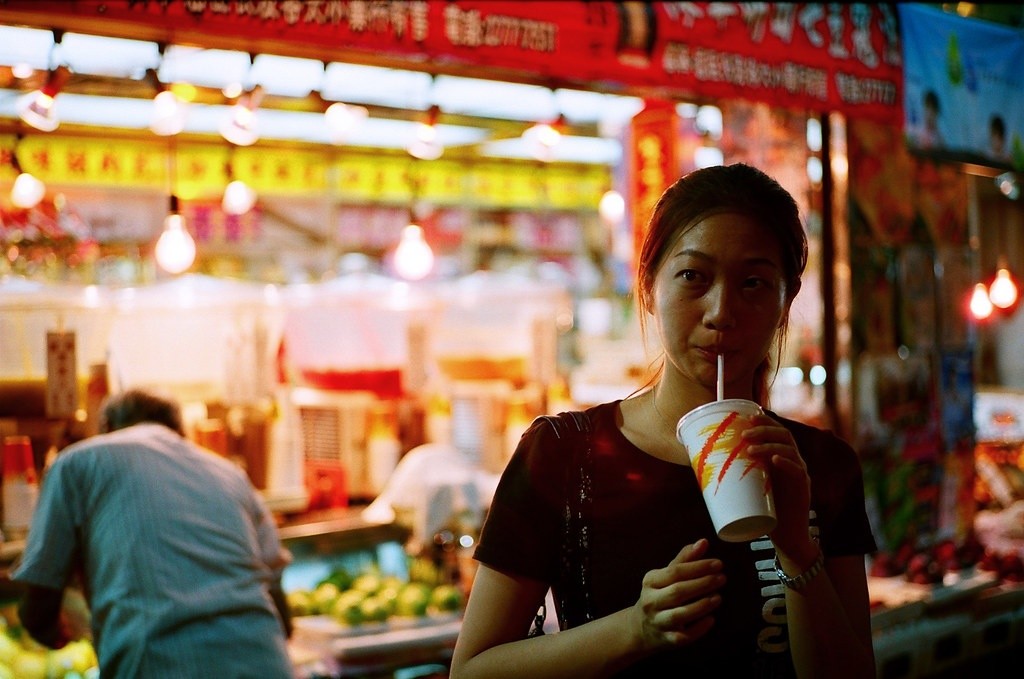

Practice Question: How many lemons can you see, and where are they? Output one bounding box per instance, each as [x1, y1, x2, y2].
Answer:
[0, 615, 99, 679]
[284, 569, 464, 626]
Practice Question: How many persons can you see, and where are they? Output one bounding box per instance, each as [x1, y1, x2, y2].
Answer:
[11, 386, 293, 679]
[448, 165, 878, 679]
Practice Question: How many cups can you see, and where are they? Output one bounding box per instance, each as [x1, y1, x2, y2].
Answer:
[194, 428, 226, 456]
[3, 436, 38, 527]
[676, 399, 775, 541]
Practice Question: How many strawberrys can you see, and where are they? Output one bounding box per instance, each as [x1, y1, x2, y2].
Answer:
[872, 539, 1024, 586]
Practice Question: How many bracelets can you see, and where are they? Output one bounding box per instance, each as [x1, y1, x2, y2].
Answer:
[773, 541, 825, 588]
